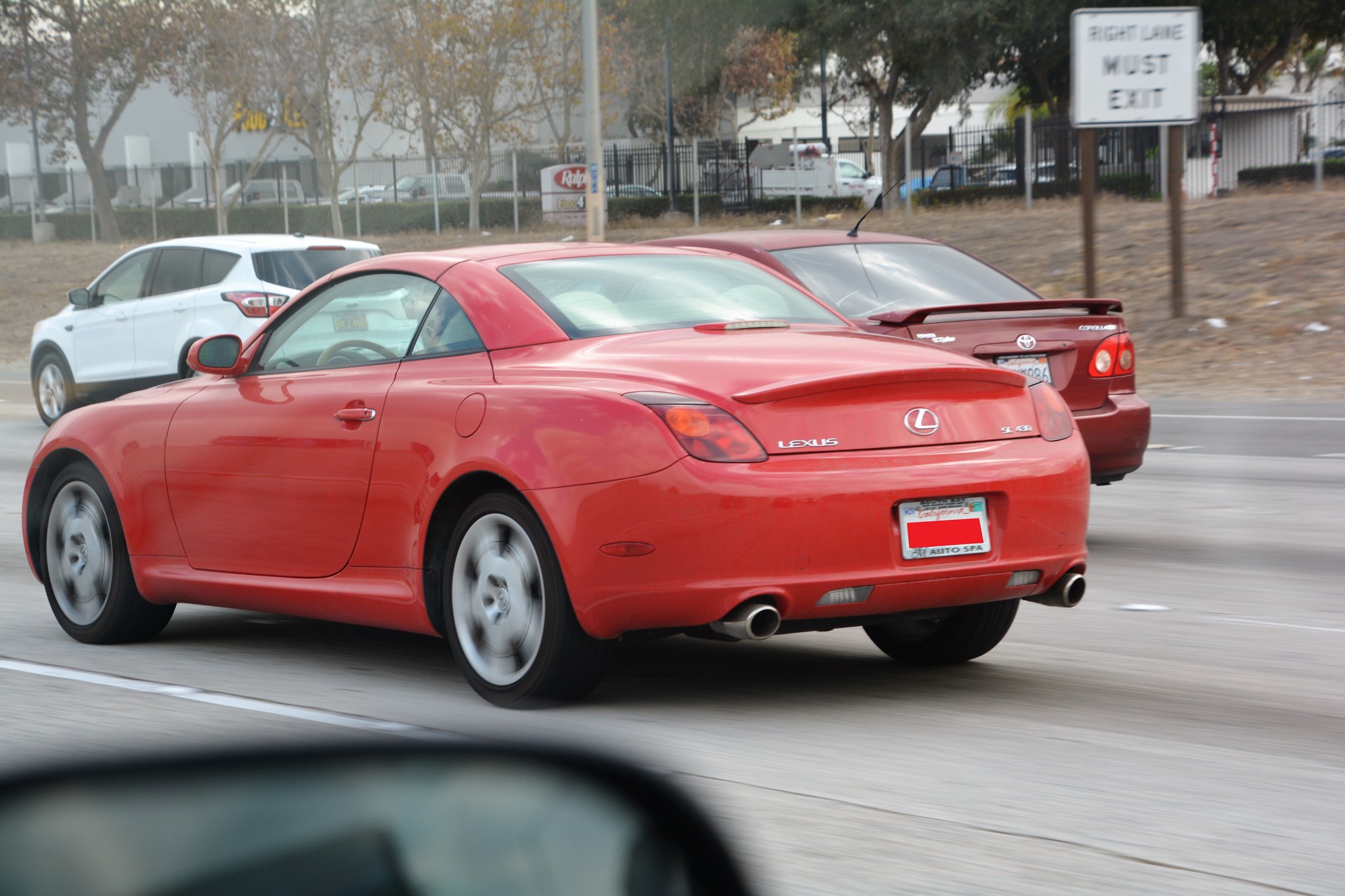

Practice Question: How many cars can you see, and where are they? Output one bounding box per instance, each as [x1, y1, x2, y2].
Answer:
[605, 183, 662, 198]
[0, 172, 541, 215]
[612, 226, 1151, 487]
[29, 233, 425, 428]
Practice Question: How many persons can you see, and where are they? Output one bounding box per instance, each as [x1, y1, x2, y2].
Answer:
[410, 296, 460, 356]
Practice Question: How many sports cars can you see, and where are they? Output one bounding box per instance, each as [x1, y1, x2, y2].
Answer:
[20, 238, 1090, 713]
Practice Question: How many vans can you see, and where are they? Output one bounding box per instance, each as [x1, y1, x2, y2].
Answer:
[701, 141, 1127, 211]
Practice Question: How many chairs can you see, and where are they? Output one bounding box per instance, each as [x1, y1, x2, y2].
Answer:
[437, 309, 486, 354]
